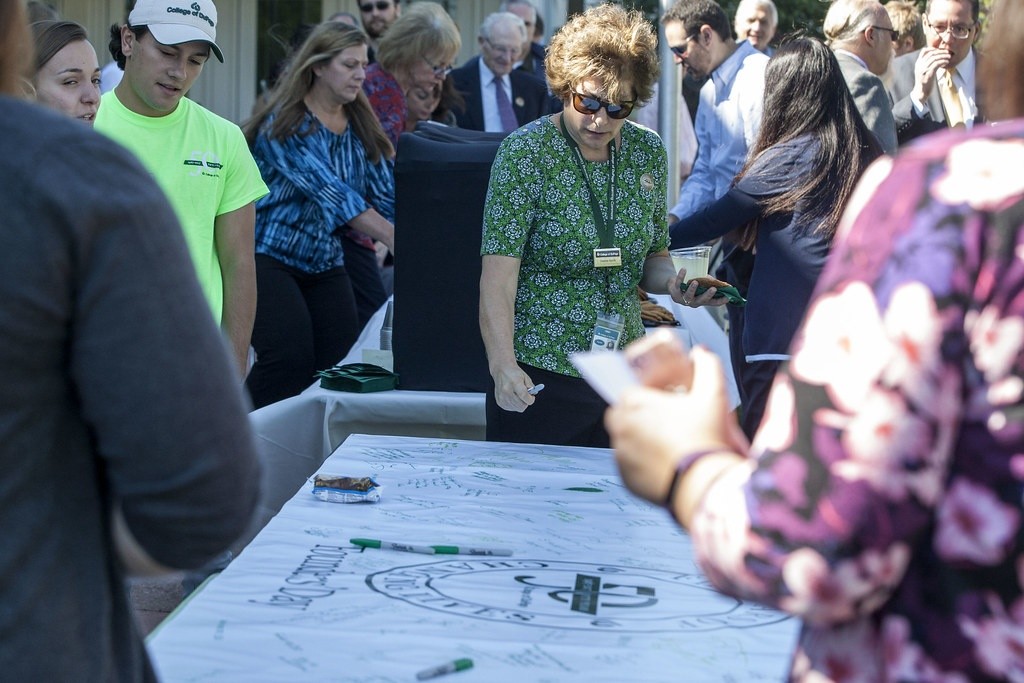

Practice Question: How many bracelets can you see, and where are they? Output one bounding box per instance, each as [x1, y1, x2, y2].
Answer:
[665, 447, 739, 523]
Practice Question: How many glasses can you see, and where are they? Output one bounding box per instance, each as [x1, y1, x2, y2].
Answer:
[485, 38, 521, 55]
[567, 82, 637, 119]
[925, 14, 977, 39]
[421, 54, 452, 76]
[670, 23, 713, 55]
[361, 2, 388, 12]
[861, 24, 900, 40]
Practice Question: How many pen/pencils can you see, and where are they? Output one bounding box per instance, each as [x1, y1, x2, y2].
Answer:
[348, 538, 436, 555]
[527, 384, 545, 395]
[432, 545, 513, 556]
[416, 659, 473, 680]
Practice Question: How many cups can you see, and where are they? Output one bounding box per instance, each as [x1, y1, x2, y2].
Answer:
[380, 301, 393, 350]
[668, 246, 712, 284]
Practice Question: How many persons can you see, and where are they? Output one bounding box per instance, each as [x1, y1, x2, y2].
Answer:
[0, 0, 264, 683]
[17, 0, 566, 412]
[601, 0, 1024, 683]
[660, 0, 983, 449]
[478, 5, 732, 448]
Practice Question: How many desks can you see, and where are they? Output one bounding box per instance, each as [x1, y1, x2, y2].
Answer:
[138, 433, 802, 683]
[325, 257, 684, 461]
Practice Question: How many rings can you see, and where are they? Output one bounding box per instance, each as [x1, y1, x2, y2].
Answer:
[682, 295, 692, 305]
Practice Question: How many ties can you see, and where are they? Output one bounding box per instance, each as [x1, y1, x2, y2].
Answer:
[943, 65, 963, 127]
[492, 77, 519, 132]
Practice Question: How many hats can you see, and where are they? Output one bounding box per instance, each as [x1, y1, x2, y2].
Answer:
[128, 0, 224, 62]
